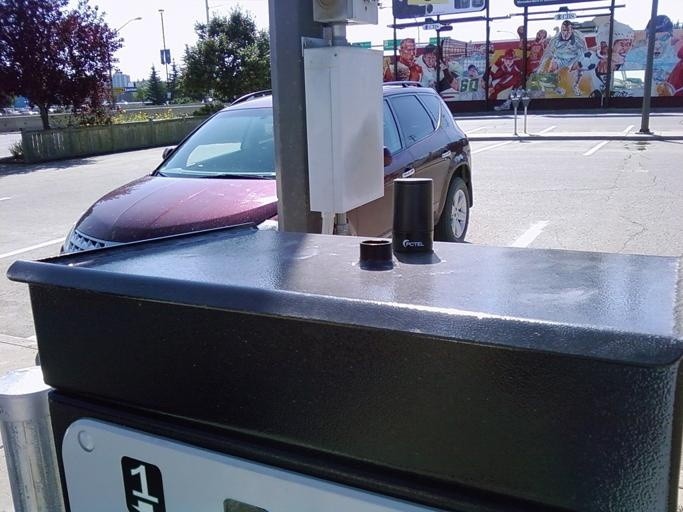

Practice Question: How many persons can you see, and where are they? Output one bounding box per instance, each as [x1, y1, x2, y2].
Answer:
[384, 14, 682, 99]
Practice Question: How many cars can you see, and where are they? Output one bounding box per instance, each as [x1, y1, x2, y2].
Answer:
[60, 81, 473, 260]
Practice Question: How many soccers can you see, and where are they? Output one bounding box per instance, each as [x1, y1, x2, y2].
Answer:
[578, 50, 599, 70]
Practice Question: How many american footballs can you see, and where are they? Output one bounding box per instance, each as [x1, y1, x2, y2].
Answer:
[517, 26, 525, 36]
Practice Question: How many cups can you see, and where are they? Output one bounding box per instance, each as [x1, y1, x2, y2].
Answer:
[394, 177, 432, 254]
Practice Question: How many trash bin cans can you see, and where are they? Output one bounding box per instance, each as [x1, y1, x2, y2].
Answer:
[0, 365, 66, 512]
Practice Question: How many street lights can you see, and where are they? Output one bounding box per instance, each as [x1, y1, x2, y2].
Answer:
[105, 16, 143, 109]
[158, 8, 168, 79]
